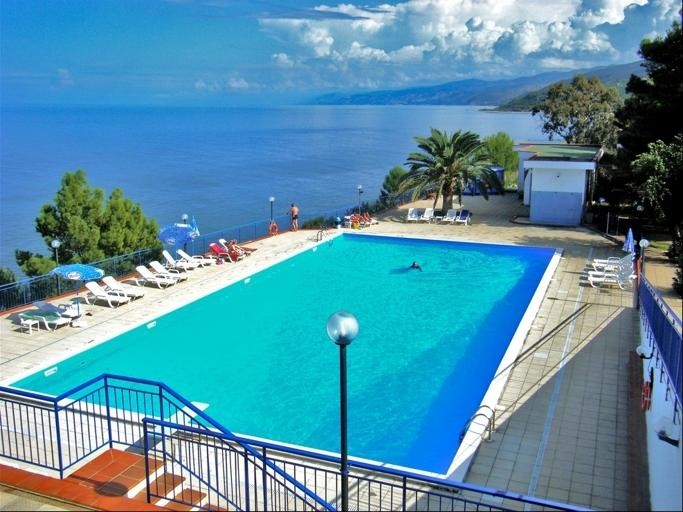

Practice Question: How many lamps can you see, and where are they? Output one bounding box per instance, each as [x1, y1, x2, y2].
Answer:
[635, 344, 653, 359]
[653, 415, 679, 448]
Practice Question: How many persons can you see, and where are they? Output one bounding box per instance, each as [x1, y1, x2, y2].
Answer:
[410, 261, 425, 272]
[227, 240, 244, 251]
[287, 203, 300, 231]
[230, 239, 237, 252]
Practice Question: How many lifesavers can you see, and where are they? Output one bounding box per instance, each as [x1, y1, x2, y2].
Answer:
[641, 381, 651, 412]
[269, 224, 280, 235]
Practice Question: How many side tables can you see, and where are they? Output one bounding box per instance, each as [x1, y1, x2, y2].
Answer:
[435, 215, 443, 223]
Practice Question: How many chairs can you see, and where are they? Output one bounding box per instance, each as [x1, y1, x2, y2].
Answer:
[441, 208, 473, 226]
[587, 253, 638, 292]
[342, 213, 378, 229]
[132, 248, 218, 290]
[406, 207, 419, 223]
[82, 276, 147, 309]
[18, 298, 94, 333]
[208, 237, 252, 263]
[418, 207, 436, 224]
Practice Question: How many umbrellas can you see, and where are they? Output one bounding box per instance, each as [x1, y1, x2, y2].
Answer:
[52, 262, 105, 318]
[158, 222, 198, 259]
[621, 228, 634, 254]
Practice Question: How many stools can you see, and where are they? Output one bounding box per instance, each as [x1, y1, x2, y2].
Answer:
[336, 224, 342, 229]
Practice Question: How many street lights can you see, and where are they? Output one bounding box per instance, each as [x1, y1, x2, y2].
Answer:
[637, 237, 650, 272]
[268, 196, 274, 222]
[636, 205, 644, 229]
[357, 183, 362, 215]
[323, 310, 360, 512]
[181, 212, 188, 254]
[50, 239, 61, 296]
